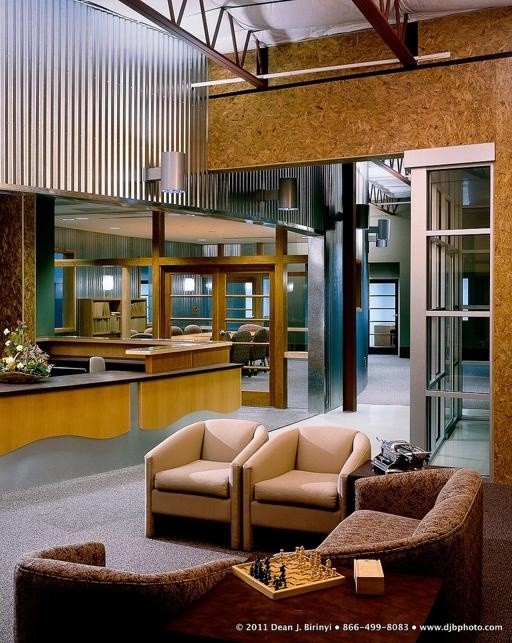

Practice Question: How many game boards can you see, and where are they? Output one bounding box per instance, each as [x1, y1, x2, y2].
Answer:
[231, 553, 346, 600]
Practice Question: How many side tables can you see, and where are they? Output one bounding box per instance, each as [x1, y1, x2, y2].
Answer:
[164, 551, 442, 643]
[347, 459, 458, 513]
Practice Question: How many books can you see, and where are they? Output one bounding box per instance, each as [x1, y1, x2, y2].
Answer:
[92, 301, 146, 336]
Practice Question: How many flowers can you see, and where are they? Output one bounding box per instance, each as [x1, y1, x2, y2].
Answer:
[0, 320, 55, 377]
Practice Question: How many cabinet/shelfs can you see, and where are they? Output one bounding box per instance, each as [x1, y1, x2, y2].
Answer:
[77, 299, 146, 338]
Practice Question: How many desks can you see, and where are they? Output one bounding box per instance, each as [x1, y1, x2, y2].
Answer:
[171, 331, 238, 341]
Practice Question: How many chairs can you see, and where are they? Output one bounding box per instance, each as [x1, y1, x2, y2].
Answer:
[315, 467, 484, 641]
[210, 324, 270, 378]
[131, 325, 203, 338]
[145, 418, 371, 551]
[12, 542, 241, 642]
[86, 356, 106, 373]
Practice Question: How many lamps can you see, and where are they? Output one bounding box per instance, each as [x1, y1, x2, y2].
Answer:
[143, 152, 185, 194]
[335, 204, 390, 248]
[256, 177, 298, 211]
